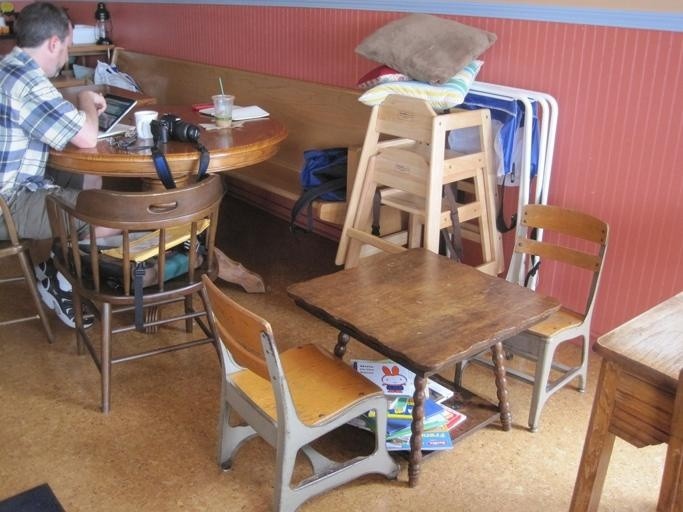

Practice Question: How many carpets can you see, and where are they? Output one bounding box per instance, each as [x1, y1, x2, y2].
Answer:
[0, 480, 63, 511]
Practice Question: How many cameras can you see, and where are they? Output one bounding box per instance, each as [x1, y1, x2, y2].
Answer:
[150, 114, 200, 144]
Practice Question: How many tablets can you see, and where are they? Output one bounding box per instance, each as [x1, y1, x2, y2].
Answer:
[98, 93, 138, 134]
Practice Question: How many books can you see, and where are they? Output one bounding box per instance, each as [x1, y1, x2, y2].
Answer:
[198, 104, 271, 122]
[346, 357, 467, 450]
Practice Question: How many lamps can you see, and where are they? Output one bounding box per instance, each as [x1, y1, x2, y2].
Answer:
[95, 1, 114, 46]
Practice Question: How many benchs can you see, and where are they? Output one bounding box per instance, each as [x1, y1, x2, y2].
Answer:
[105, 46, 410, 258]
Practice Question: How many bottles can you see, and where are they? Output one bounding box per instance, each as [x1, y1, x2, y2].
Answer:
[93, 3, 112, 46]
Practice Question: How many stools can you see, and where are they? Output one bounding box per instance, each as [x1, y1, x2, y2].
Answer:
[335, 94, 506, 276]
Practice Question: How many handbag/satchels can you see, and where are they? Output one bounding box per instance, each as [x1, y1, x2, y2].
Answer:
[444, 93, 539, 233]
[302, 149, 346, 202]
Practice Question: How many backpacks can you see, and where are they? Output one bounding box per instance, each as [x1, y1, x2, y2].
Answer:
[51, 236, 204, 290]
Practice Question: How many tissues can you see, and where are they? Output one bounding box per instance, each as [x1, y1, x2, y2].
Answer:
[70, 23, 100, 45]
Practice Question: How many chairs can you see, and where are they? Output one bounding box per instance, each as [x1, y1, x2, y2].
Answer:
[454, 205, 610, 433]
[656, 369, 683, 512]
[201, 274, 401, 511]
[47, 173, 226, 413]
[0, 194, 55, 344]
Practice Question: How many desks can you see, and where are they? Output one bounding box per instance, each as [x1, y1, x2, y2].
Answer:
[568, 290, 683, 512]
[49, 44, 289, 192]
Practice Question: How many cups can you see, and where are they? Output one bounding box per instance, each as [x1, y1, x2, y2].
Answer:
[134, 110, 158, 138]
[211, 93, 235, 127]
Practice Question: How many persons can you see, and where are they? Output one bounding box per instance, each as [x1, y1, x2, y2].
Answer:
[0, 1, 108, 331]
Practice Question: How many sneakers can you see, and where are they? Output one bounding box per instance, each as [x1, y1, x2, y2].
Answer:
[34, 258, 53, 280]
[36, 270, 95, 329]
